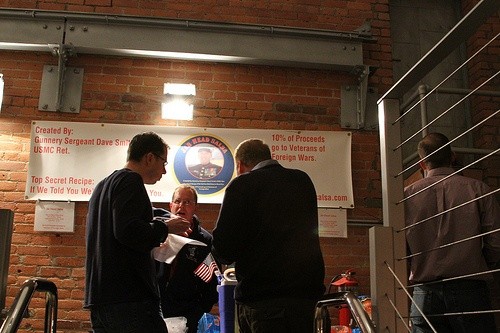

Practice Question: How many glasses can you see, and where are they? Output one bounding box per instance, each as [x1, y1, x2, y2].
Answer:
[173, 199, 198, 207]
[145, 151, 168, 166]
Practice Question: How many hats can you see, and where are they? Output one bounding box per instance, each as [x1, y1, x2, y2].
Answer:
[198, 148, 213, 153]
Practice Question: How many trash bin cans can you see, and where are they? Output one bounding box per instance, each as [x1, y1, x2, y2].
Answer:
[216, 268, 238, 333]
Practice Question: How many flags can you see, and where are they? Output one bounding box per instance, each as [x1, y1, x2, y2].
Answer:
[194, 252, 219, 284]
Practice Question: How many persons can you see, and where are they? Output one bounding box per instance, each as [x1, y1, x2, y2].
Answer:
[400, 132, 500, 333]
[188, 148, 222, 179]
[83, 131, 194, 333]
[155, 183, 220, 333]
[209, 138, 325, 333]
